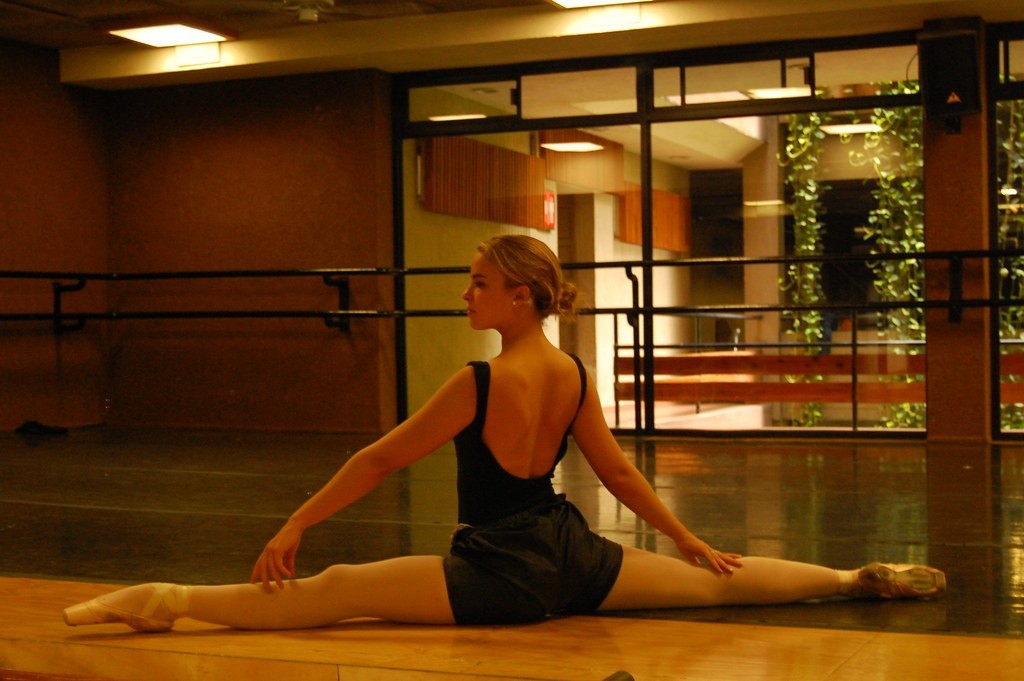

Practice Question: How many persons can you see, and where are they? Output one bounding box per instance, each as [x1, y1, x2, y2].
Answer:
[63, 234, 946, 632]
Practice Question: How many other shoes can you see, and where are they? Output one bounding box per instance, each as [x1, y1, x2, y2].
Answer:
[64, 576, 189, 631]
[839, 559, 947, 602]
